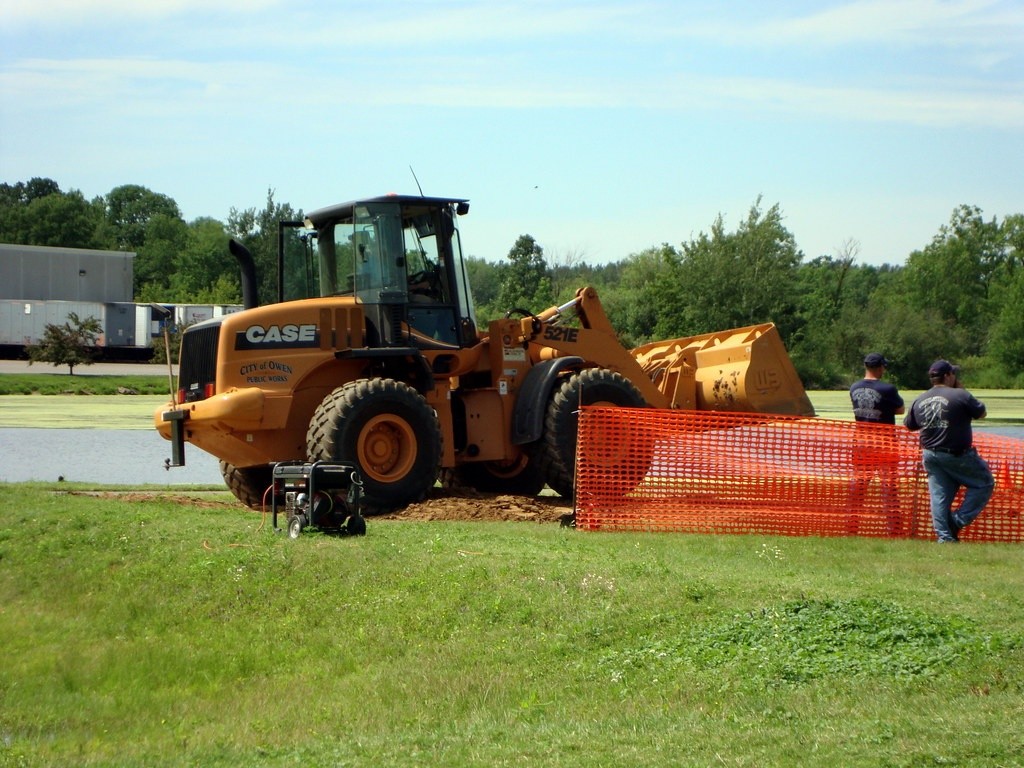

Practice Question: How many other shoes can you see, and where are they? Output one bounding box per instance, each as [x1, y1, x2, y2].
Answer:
[946, 512, 960, 542]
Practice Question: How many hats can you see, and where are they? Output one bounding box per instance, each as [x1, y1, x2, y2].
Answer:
[928, 360, 959, 377]
[864, 353, 888, 369]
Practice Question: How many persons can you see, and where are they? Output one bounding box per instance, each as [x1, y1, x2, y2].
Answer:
[903, 360, 995, 543]
[847, 353, 906, 538]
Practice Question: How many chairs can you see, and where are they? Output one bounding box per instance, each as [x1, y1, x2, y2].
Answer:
[409, 263, 442, 302]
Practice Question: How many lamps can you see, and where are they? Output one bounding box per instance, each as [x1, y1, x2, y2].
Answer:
[79, 269, 85, 276]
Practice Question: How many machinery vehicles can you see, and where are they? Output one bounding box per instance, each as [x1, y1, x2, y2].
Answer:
[151, 193, 820, 517]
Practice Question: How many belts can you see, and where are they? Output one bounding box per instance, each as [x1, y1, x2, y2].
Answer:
[928, 447, 950, 453]
